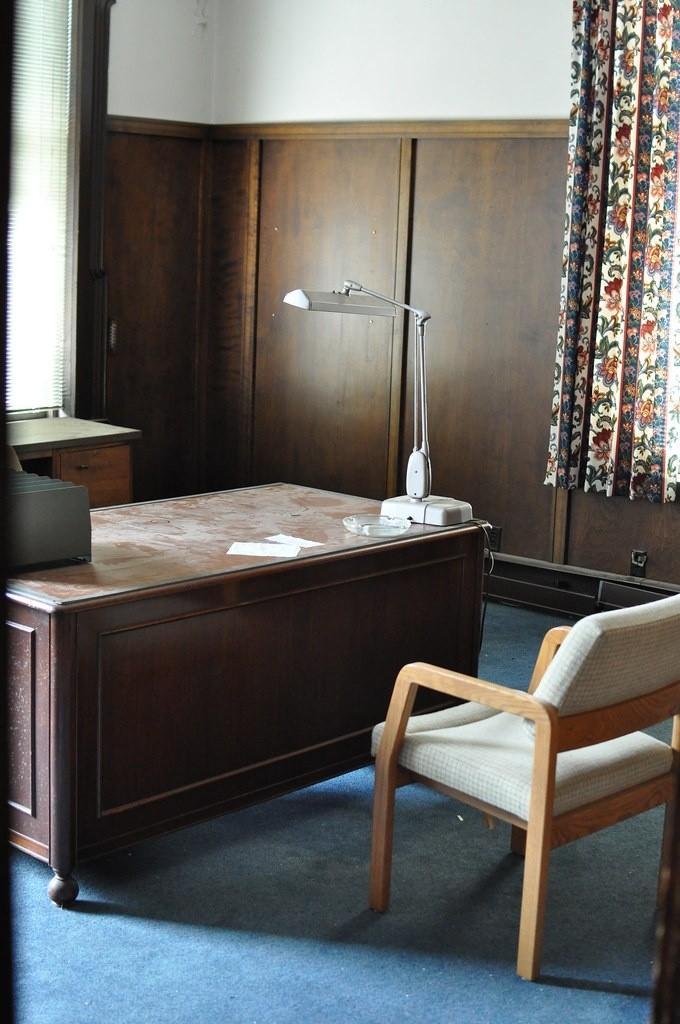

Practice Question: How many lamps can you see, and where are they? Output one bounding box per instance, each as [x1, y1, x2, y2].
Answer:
[283, 279, 473, 528]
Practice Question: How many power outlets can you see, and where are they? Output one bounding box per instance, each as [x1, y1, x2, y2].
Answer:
[485, 525, 502, 552]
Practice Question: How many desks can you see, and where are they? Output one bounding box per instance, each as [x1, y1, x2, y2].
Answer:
[5, 417, 142, 510]
[6, 484, 493, 909]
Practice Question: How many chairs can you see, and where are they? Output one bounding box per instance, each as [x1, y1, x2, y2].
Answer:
[368, 592, 680, 983]
